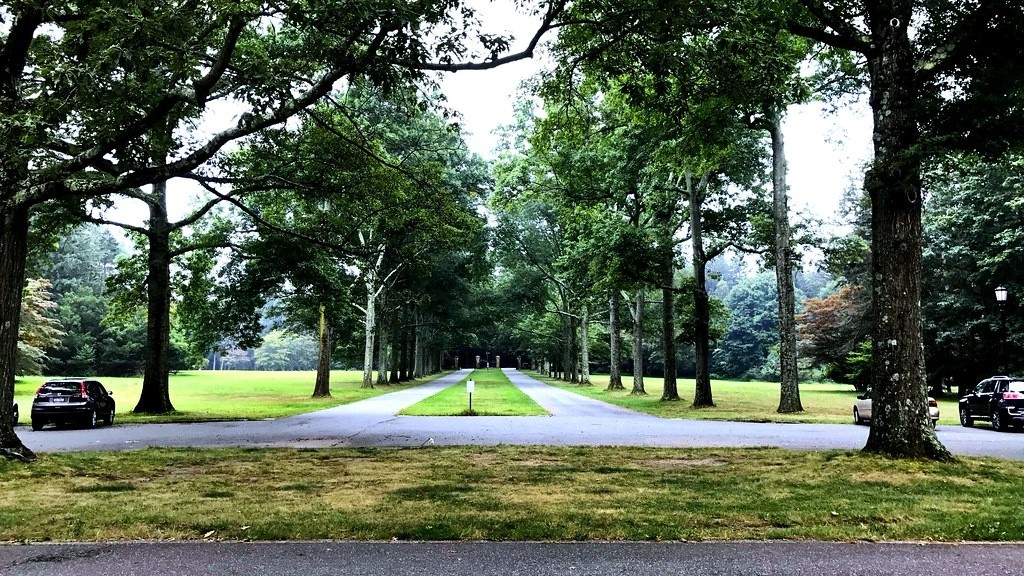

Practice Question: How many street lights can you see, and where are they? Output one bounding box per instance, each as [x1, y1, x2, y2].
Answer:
[995, 282, 1008, 374]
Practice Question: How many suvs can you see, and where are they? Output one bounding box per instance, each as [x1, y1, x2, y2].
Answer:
[958, 375, 1024, 432]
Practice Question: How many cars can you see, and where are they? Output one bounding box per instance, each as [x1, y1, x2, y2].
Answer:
[13, 399, 19, 427]
[30, 378, 115, 431]
[852, 386, 939, 428]
